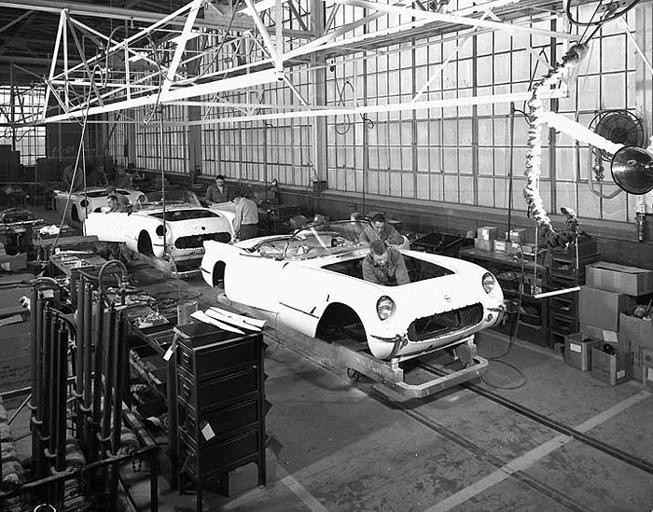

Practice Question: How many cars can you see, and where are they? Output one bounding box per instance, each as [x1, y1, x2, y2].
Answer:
[82, 189, 239, 262]
[199, 219, 505, 363]
[52, 186, 148, 223]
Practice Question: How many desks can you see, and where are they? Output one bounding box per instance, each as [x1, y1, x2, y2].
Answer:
[459, 246, 548, 351]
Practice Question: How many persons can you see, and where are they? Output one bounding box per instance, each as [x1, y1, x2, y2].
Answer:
[105, 186, 130, 207]
[106, 195, 126, 213]
[365, 214, 389, 244]
[205, 176, 231, 206]
[230, 191, 259, 241]
[361, 237, 410, 288]
[62, 161, 83, 194]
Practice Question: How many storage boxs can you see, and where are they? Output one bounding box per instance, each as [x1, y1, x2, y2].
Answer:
[470, 221, 536, 258]
[563, 259, 652, 387]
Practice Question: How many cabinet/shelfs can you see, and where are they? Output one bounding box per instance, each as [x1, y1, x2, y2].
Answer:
[544, 234, 599, 356]
[174, 324, 270, 512]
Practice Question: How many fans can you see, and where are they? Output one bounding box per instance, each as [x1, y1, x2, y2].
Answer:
[608, 144, 652, 197]
[584, 107, 646, 166]
